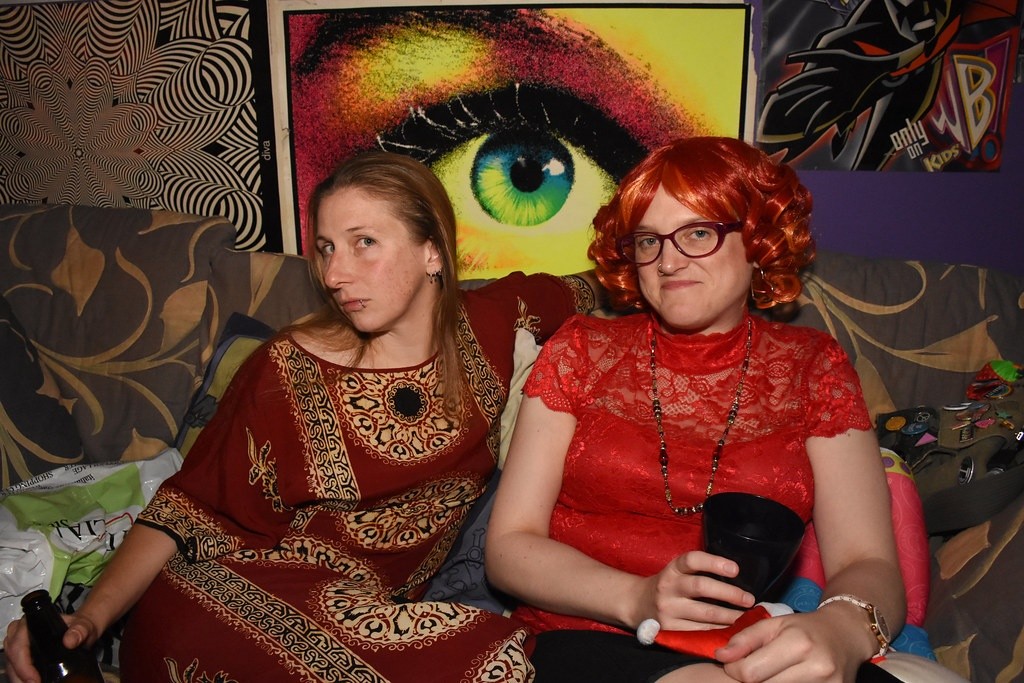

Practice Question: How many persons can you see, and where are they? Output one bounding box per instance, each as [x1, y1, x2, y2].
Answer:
[487, 135, 908, 683]
[3, 149, 610, 683]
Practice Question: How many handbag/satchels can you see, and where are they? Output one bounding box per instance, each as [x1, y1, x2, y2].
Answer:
[874, 406, 939, 461]
[906, 385, 1024, 561]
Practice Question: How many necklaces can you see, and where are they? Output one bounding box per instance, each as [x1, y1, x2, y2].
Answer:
[649, 316, 753, 515]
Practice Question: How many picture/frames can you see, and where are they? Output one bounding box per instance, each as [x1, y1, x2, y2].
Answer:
[249, 0, 764, 278]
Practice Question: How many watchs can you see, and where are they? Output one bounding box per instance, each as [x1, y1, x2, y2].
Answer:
[816, 593, 892, 658]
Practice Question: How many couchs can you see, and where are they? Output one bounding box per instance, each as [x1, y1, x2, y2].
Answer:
[0, 206, 1024, 683]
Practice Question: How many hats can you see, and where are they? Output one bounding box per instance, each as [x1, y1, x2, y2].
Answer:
[637, 603, 795, 659]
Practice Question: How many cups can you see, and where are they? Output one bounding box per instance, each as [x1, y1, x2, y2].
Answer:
[696, 492, 807, 613]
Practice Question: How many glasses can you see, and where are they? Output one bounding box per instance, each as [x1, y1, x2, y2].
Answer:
[617, 221, 745, 266]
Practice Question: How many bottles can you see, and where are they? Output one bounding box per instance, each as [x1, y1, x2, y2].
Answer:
[21, 589, 106, 683]
